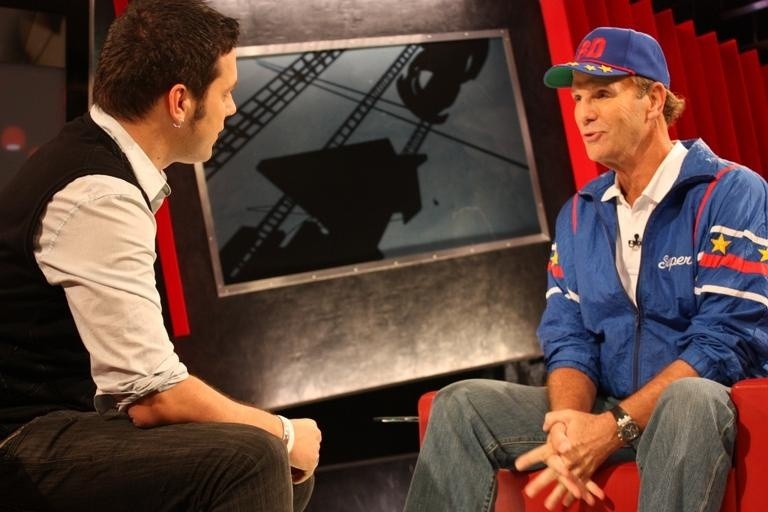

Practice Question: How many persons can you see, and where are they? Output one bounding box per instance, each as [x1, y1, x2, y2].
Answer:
[0, 1, 323, 511]
[402, 24, 768, 512]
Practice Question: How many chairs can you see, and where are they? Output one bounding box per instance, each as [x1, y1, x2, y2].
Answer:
[418, 379, 768, 512]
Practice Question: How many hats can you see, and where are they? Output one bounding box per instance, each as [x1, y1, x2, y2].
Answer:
[543, 28, 670, 90]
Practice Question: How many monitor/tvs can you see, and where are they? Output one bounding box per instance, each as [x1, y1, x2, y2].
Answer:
[0, 0, 95, 190]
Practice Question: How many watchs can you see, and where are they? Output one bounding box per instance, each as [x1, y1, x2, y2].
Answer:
[609, 404, 641, 443]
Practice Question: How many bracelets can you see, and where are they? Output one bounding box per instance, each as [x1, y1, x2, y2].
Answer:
[277, 415, 295, 453]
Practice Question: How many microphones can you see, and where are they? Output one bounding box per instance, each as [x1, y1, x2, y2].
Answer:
[628, 233, 642, 247]
